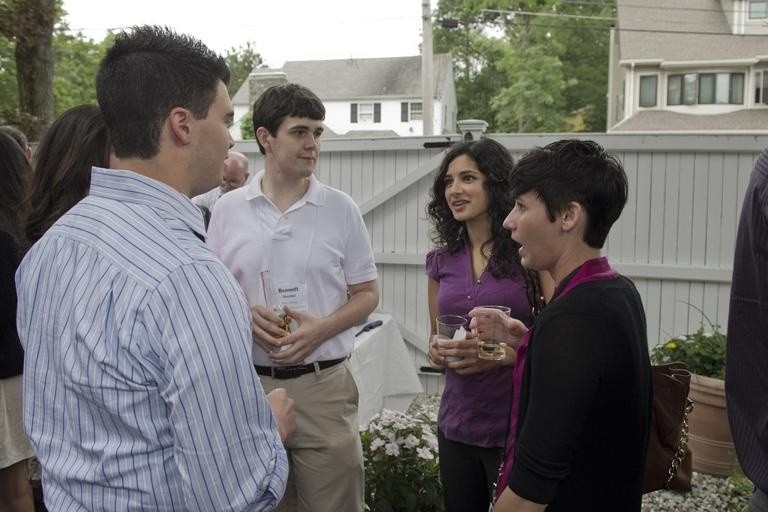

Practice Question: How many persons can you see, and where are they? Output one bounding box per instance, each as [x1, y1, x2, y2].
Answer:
[21, 104, 114, 245]
[481, 137, 657, 511]
[421, 136, 558, 510]
[723, 146, 768, 510]
[184, 151, 250, 237]
[12, 22, 303, 510]
[0, 122, 33, 166]
[1, 126, 52, 512]
[203, 79, 385, 512]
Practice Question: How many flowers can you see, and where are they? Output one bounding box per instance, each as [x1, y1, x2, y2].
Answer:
[648, 300, 726, 381]
[356, 395, 445, 512]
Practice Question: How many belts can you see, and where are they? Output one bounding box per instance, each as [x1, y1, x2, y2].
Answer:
[252, 356, 350, 379]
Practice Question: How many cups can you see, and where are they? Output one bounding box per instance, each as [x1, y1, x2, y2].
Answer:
[435, 314, 469, 366]
[474, 304, 513, 361]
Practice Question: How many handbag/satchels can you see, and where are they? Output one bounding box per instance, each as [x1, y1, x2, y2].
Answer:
[641, 362, 694, 495]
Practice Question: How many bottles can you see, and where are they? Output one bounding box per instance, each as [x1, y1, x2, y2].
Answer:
[259, 270, 292, 353]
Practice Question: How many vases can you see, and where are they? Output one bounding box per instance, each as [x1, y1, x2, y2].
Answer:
[682, 374, 735, 476]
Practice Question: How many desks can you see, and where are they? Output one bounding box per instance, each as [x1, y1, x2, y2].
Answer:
[346, 313, 424, 431]
